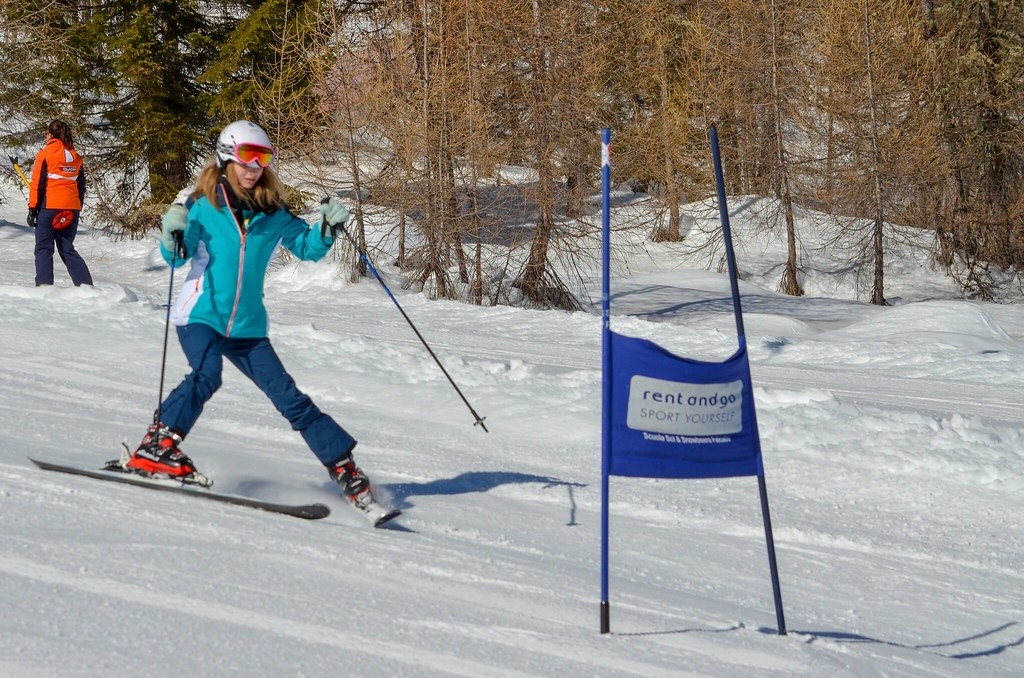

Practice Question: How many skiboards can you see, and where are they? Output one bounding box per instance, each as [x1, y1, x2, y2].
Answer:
[24, 452, 406, 530]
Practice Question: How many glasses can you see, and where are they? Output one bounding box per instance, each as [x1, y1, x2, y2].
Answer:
[235, 143, 275, 168]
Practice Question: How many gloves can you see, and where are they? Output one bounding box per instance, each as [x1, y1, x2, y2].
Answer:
[318, 198, 349, 237]
[162, 204, 189, 251]
[27, 207, 38, 227]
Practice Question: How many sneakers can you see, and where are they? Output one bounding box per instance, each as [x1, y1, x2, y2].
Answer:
[128, 423, 194, 479]
[328, 452, 372, 506]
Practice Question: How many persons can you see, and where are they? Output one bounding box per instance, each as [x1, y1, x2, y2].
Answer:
[126, 120, 401, 523]
[26, 118, 95, 288]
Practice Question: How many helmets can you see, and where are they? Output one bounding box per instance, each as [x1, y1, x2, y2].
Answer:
[216, 119, 272, 168]
